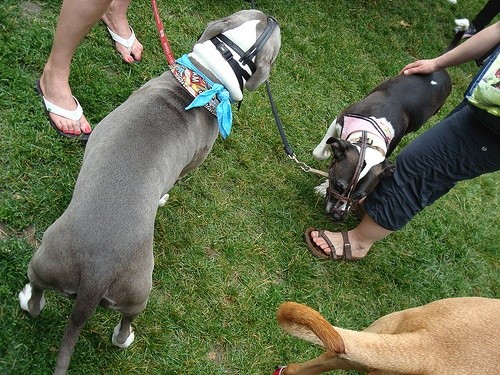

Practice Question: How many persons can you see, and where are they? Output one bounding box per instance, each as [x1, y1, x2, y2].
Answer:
[37, 0, 143, 139]
[454, 0, 500, 66]
[305, 21, 500, 262]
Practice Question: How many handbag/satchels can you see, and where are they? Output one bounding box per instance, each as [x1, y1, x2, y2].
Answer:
[462, 42, 500, 119]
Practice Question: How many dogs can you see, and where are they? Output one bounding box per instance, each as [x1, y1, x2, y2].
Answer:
[18, 10, 283, 375]
[270, 294, 500, 375]
[310, 68, 452, 222]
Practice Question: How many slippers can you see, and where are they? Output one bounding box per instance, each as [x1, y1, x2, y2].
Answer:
[106, 24, 145, 66]
[304, 225, 357, 264]
[37, 78, 93, 143]
